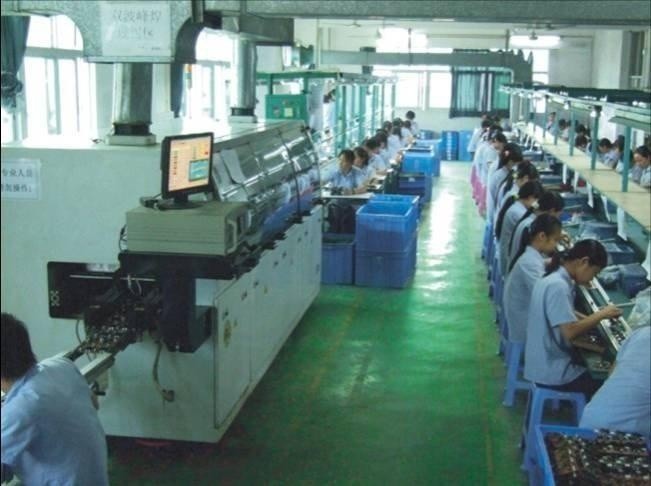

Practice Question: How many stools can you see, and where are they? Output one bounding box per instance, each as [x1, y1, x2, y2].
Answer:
[503, 344, 535, 408]
[519, 385, 586, 471]
[480, 225, 523, 354]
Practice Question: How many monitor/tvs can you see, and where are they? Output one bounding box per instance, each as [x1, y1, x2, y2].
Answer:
[155, 132, 214, 210]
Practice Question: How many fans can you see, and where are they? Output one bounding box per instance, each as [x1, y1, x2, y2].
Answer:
[318, 18, 391, 31]
[513, 23, 576, 32]
[510, 28, 549, 41]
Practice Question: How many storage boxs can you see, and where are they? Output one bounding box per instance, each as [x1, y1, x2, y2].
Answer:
[401, 151, 433, 175]
[356, 201, 414, 253]
[534, 424, 649, 481]
[358, 238, 417, 289]
[420, 129, 474, 160]
[322, 232, 355, 286]
[400, 181, 432, 206]
[372, 194, 421, 233]
[414, 140, 438, 156]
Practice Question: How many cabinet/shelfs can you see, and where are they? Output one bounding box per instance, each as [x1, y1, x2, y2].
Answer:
[212, 204, 323, 444]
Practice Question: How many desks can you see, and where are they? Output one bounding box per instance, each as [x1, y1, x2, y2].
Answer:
[321, 130, 418, 200]
[501, 128, 651, 360]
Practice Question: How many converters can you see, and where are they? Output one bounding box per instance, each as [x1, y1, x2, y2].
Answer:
[139, 196, 159, 208]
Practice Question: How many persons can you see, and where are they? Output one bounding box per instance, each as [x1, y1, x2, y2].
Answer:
[0, 312, 109, 486]
[325, 110, 421, 233]
